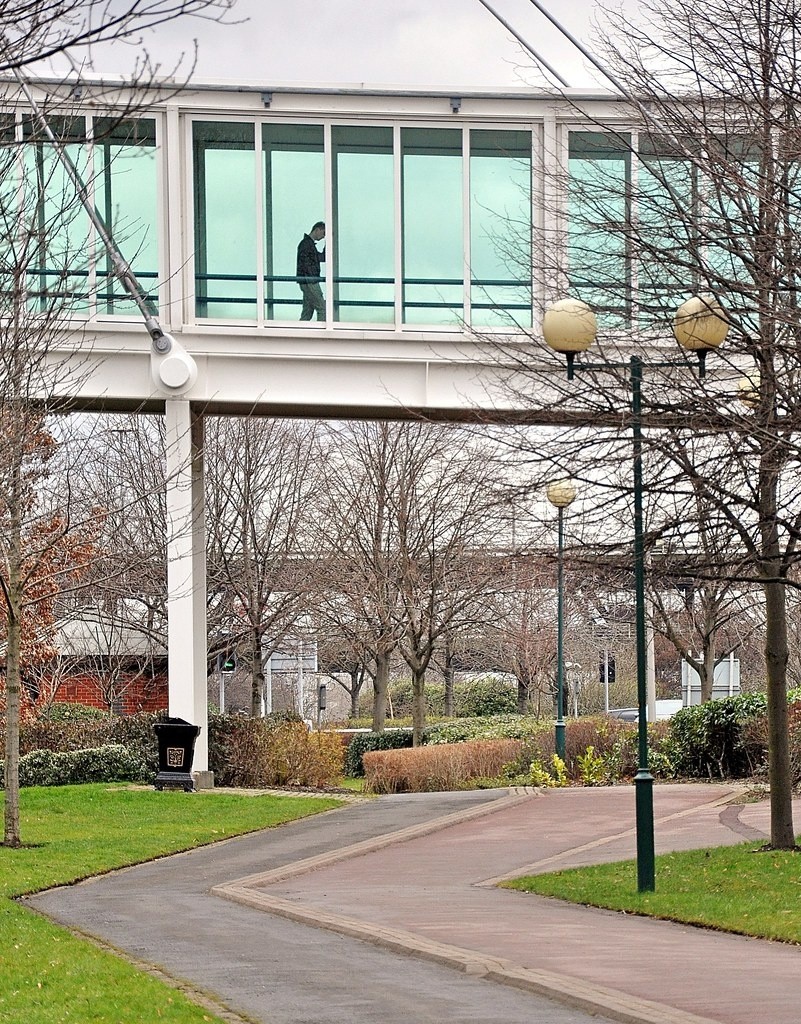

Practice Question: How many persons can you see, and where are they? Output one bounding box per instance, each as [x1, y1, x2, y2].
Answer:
[297, 222, 327, 320]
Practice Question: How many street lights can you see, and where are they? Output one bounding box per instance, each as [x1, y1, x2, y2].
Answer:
[546, 479, 576, 760]
[542, 287, 730, 890]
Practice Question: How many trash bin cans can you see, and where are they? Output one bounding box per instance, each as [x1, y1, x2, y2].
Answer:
[152, 715, 202, 792]
